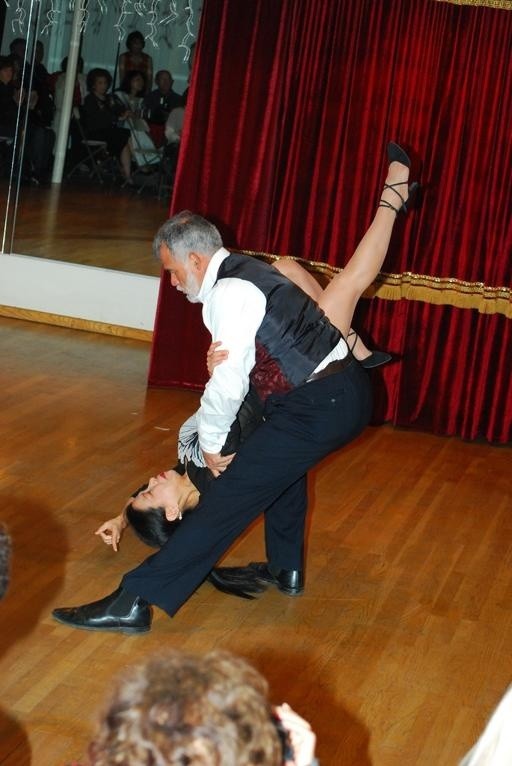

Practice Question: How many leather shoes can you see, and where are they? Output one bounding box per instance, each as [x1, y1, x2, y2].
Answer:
[52, 588, 152, 633]
[249, 562, 304, 596]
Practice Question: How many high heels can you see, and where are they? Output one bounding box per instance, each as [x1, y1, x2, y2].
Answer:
[347, 331, 393, 369]
[379, 141, 419, 215]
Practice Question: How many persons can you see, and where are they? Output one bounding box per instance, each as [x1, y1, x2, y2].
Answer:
[92, 140, 422, 599]
[1, 28, 198, 198]
[50, 209, 384, 635]
[88, 647, 323, 765]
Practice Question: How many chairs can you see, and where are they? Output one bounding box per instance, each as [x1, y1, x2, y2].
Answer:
[65, 103, 106, 184]
[136, 138, 176, 201]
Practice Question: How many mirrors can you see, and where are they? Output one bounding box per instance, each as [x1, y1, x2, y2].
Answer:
[2, 0, 203, 279]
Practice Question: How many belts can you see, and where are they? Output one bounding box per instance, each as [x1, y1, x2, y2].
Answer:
[305, 352, 353, 383]
